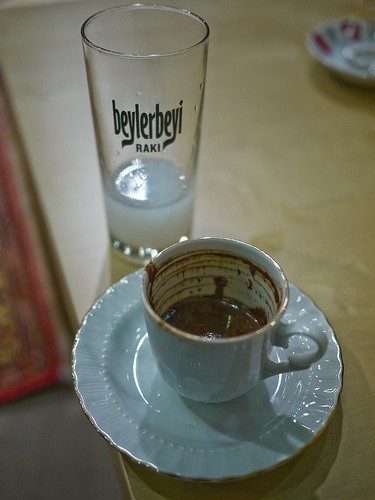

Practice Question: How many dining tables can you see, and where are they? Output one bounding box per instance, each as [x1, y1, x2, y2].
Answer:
[1, 1, 374, 500]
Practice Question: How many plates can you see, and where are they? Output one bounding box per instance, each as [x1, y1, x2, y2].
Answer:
[71, 264, 344, 486]
[301, 13, 375, 86]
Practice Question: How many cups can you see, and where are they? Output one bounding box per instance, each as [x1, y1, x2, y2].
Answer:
[79, 2, 210, 266]
[138, 236, 330, 404]
